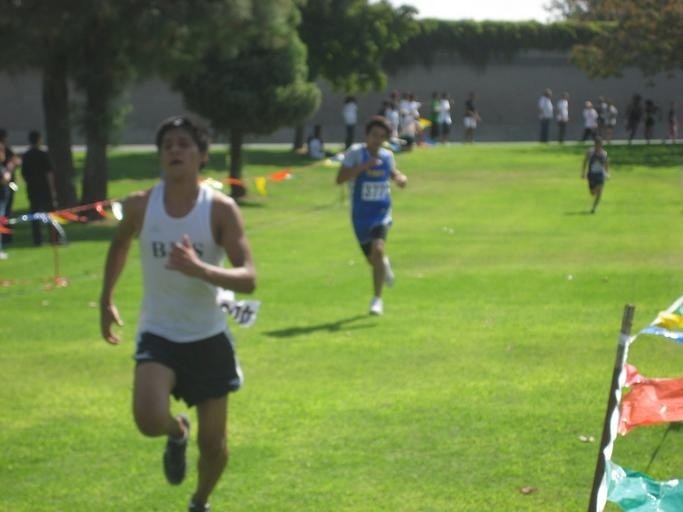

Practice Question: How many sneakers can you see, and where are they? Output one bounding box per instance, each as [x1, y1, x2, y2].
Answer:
[369, 297, 384, 315]
[382, 254, 396, 287]
[188, 495, 210, 511]
[163, 413, 191, 485]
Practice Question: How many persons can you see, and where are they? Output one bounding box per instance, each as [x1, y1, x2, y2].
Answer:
[556, 91, 570, 142]
[538, 88, 554, 142]
[623, 95, 644, 144]
[641, 99, 664, 143]
[583, 138, 609, 213]
[580, 96, 618, 145]
[666, 101, 680, 144]
[342, 96, 358, 149]
[379, 90, 453, 148]
[463, 93, 481, 144]
[0, 130, 59, 259]
[337, 114, 408, 315]
[100, 114, 256, 512]
[308, 122, 334, 159]
